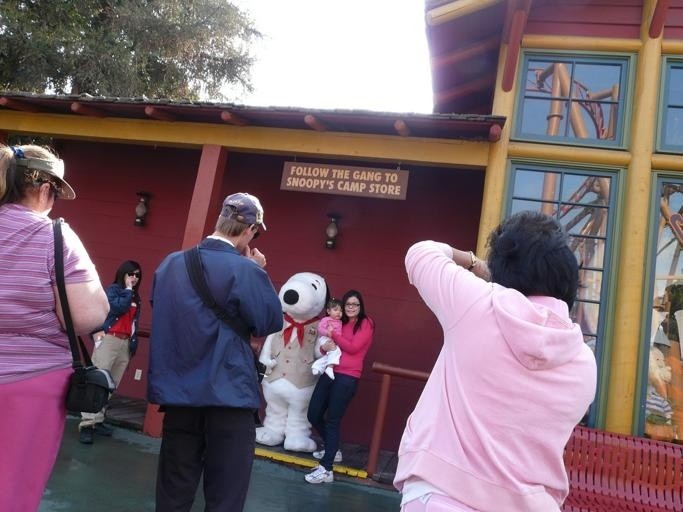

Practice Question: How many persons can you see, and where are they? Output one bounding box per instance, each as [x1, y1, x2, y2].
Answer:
[304, 290, 376, 483]
[392, 211, 597, 512]
[79, 261, 142, 444]
[311, 299, 344, 380]
[147, 192, 283, 512]
[0, 144, 110, 512]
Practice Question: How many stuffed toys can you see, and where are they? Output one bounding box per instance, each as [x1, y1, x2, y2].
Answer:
[256, 272, 329, 452]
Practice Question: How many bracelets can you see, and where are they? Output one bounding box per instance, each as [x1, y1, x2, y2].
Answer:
[469, 250, 477, 271]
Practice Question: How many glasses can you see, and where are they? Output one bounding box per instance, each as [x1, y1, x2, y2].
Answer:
[127, 272, 140, 278]
[345, 303, 361, 306]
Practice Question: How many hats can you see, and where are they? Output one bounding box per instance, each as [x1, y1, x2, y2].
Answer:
[16, 156, 76, 200]
[221, 192, 267, 231]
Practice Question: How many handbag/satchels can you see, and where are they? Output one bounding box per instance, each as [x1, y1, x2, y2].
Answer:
[66, 365, 115, 413]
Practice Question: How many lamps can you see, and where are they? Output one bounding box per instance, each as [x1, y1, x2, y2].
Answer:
[322, 211, 341, 249]
[131, 190, 153, 227]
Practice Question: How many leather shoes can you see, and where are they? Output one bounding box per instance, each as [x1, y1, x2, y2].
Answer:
[94, 422, 113, 435]
[80, 426, 93, 443]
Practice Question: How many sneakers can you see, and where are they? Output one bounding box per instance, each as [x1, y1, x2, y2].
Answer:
[313, 449, 342, 462]
[305, 465, 333, 483]
